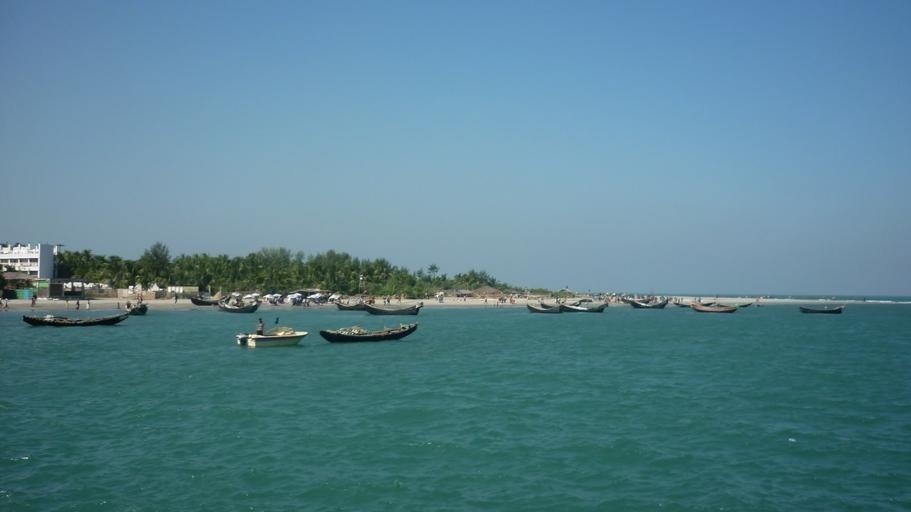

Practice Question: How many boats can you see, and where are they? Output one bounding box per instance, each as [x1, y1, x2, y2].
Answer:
[235, 331, 309, 347]
[527, 296, 753, 312]
[335, 299, 424, 315]
[321, 323, 418, 342]
[800, 305, 843, 314]
[192, 295, 263, 313]
[23, 304, 148, 326]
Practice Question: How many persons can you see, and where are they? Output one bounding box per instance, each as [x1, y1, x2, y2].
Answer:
[399, 295, 401, 304]
[388, 295, 391, 305]
[499, 295, 507, 303]
[383, 294, 387, 306]
[126, 301, 132, 311]
[4, 297, 10, 307]
[464, 293, 467, 302]
[510, 296, 513, 303]
[31, 297, 35, 308]
[75, 298, 81, 311]
[1, 296, 2, 306]
[86, 300, 90, 311]
[33, 294, 37, 302]
[427, 294, 430, 299]
[174, 294, 178, 304]
[372, 296, 376, 305]
[485, 297, 488, 303]
[599, 291, 684, 307]
[275, 317, 281, 327]
[64, 297, 70, 309]
[256, 318, 265, 335]
